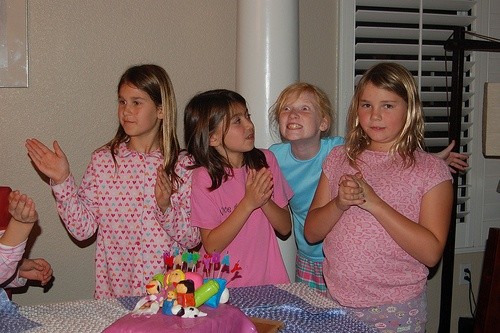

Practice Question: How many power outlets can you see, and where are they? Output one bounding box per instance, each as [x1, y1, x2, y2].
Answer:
[458, 263, 473, 287]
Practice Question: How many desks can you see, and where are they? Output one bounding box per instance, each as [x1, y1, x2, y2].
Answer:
[0, 283, 381, 332]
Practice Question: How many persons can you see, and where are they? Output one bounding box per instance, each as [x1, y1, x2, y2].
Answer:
[303, 62, 455, 333]
[25, 64, 201, 301]
[267, 82, 469, 300]
[0, 190, 54, 307]
[183, 88, 295, 288]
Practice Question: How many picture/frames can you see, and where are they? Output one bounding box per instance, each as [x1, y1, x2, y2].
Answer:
[0, 0, 30, 88]
